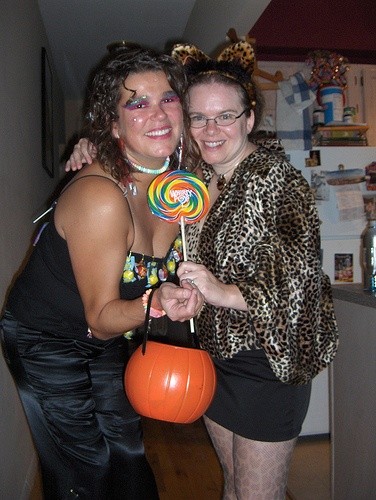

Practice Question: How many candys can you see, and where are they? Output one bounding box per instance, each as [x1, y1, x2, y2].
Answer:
[146, 170, 211, 333]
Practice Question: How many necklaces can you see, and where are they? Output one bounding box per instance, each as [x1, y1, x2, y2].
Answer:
[213, 145, 248, 191]
[133, 157, 170, 174]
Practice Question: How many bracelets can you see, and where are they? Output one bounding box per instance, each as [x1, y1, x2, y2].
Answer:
[142, 289, 166, 318]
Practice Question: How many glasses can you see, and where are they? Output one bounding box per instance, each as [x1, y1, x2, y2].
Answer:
[189, 108, 247, 128]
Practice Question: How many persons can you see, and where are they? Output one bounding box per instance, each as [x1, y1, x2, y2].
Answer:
[0, 42, 204, 500]
[65, 41, 339, 500]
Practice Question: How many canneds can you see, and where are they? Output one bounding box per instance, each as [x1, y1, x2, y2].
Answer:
[343, 114, 352, 123]
[312, 106, 325, 123]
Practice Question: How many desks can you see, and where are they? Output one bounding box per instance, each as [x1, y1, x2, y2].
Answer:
[329, 283, 375, 499]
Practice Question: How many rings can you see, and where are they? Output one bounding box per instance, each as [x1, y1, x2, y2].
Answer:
[192, 281, 194, 284]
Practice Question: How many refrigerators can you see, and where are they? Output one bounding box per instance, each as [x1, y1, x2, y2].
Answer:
[285, 148, 376, 434]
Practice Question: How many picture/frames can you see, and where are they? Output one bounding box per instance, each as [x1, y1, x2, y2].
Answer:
[41, 47, 56, 178]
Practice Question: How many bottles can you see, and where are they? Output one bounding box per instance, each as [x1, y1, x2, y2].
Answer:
[313, 105, 325, 128]
[343, 107, 356, 124]
[319, 86, 344, 126]
